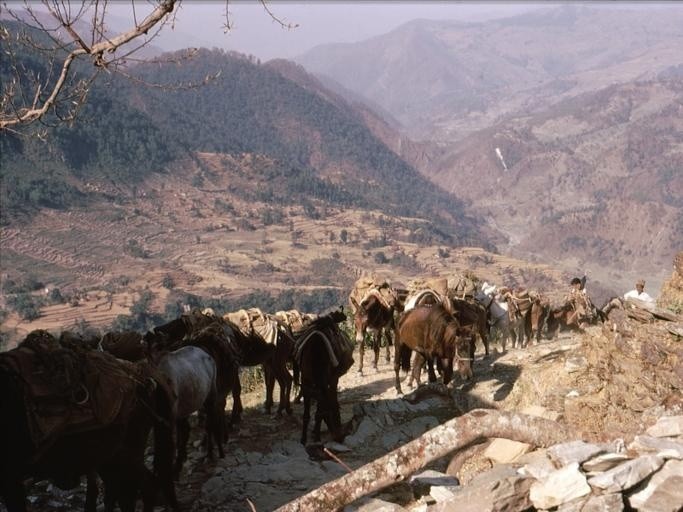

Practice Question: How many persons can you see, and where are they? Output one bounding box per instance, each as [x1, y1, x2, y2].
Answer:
[624, 279, 652, 303]
[572, 278, 587, 298]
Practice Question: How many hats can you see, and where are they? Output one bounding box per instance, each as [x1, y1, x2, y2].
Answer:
[636, 278, 645, 286]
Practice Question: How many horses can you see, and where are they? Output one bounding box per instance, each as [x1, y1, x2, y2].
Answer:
[350, 281, 550, 396]
[547, 300, 609, 341]
[2, 310, 291, 511]
[273, 308, 353, 446]
